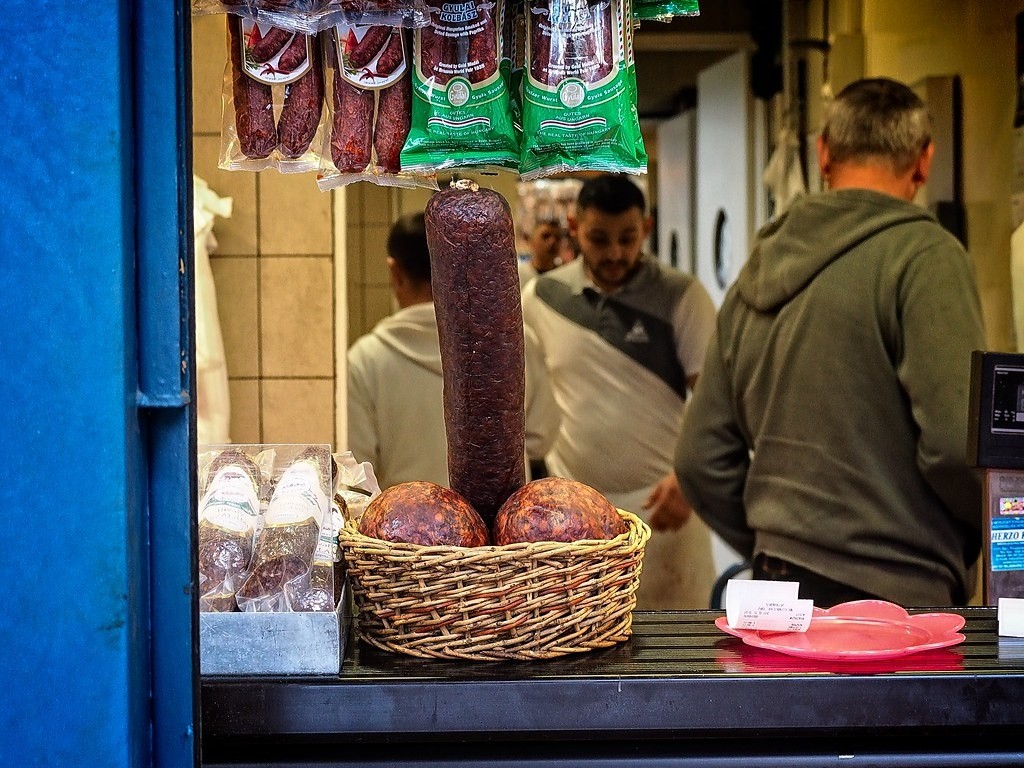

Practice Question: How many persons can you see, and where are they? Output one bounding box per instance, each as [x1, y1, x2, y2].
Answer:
[673, 76, 985, 608]
[345, 214, 560, 512]
[521, 172, 719, 613]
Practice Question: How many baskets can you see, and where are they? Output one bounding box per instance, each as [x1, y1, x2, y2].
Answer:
[338, 508, 651, 662]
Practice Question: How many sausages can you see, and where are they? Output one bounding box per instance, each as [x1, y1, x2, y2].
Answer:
[215, 0, 649, 175]
[197, 444, 346, 613]
[426, 178, 526, 534]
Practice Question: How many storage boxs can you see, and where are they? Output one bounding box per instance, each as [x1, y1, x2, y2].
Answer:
[199, 578, 349, 672]
[998, 598, 1024, 638]
[966, 350, 1024, 469]
[982, 472, 1024, 606]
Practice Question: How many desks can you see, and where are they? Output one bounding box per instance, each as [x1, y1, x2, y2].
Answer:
[199, 609, 1024, 731]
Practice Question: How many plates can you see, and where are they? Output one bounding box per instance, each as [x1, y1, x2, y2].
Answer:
[713, 599, 966, 663]
